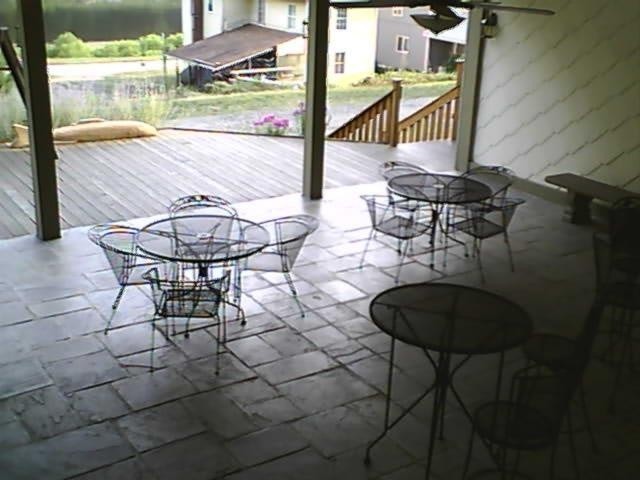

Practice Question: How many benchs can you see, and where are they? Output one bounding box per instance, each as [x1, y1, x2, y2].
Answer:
[545, 171, 637, 225]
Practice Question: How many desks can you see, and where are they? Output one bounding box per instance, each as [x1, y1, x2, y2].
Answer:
[387, 174, 489, 267]
[363, 282, 533, 476]
[135, 213, 270, 328]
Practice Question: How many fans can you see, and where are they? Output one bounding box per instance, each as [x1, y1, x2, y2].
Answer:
[327, 0, 554, 36]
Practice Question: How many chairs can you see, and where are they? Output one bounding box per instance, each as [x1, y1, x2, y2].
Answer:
[234, 214, 321, 324]
[381, 158, 444, 228]
[446, 165, 513, 240]
[359, 193, 434, 280]
[526, 306, 613, 477]
[142, 266, 236, 379]
[460, 363, 555, 477]
[89, 223, 185, 338]
[169, 194, 244, 314]
[592, 234, 640, 367]
[446, 196, 526, 282]
[607, 194, 640, 283]
